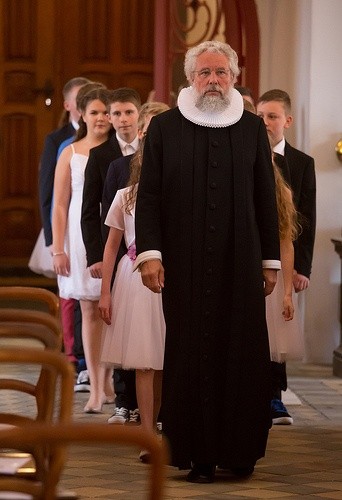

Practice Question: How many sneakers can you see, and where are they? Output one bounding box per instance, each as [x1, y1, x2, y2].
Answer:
[127, 408, 140, 424]
[74, 370, 91, 392]
[107, 407, 130, 425]
[270, 399, 294, 425]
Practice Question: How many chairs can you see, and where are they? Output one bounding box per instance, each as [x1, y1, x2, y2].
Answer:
[0, 286, 169, 500]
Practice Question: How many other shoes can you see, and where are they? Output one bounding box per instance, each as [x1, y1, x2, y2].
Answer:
[102, 396, 117, 411]
[139, 449, 152, 464]
[190, 459, 217, 483]
[83, 399, 102, 414]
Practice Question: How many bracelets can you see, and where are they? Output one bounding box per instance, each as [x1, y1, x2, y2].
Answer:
[53, 251, 65, 256]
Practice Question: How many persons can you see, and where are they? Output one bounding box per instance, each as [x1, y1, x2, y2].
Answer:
[97, 133, 166, 460]
[133, 40, 280, 483]
[236, 86, 317, 424]
[28, 77, 172, 425]
